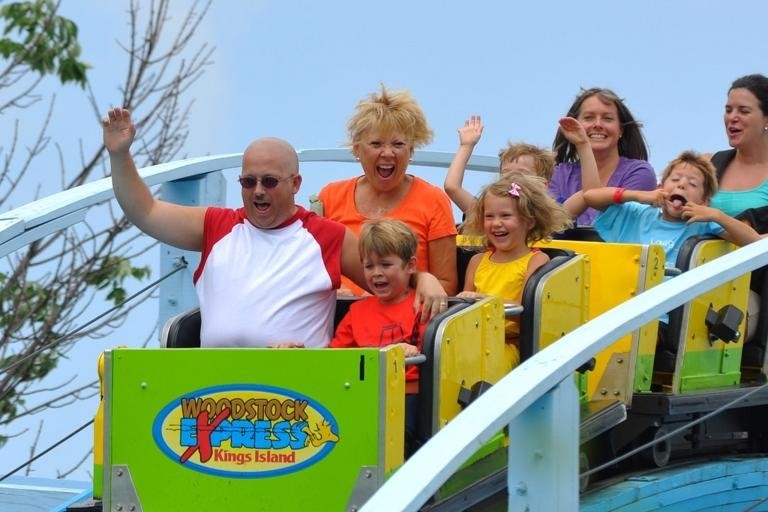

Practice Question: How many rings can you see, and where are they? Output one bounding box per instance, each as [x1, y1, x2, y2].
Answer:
[440, 303, 447, 307]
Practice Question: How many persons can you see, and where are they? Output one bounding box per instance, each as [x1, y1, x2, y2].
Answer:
[103, 108, 449, 349]
[444, 114, 602, 234]
[266, 218, 439, 454]
[582, 150, 762, 353]
[545, 85, 658, 226]
[706, 74, 767, 235]
[309, 81, 458, 297]
[456, 170, 575, 374]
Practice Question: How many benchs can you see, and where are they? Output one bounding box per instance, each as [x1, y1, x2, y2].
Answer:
[422, 244, 589, 404]
[658, 235, 753, 394]
[158, 295, 504, 466]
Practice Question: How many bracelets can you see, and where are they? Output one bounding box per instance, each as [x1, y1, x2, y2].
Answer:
[612, 187, 627, 207]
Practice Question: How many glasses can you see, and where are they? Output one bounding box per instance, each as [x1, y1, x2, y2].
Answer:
[238, 175, 301, 189]
